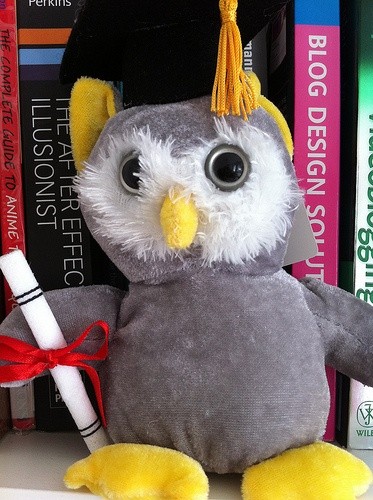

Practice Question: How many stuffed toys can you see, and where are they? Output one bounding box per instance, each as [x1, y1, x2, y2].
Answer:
[0, 0, 373, 500]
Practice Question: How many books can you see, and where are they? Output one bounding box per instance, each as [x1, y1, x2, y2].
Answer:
[0, 0, 373, 453]
